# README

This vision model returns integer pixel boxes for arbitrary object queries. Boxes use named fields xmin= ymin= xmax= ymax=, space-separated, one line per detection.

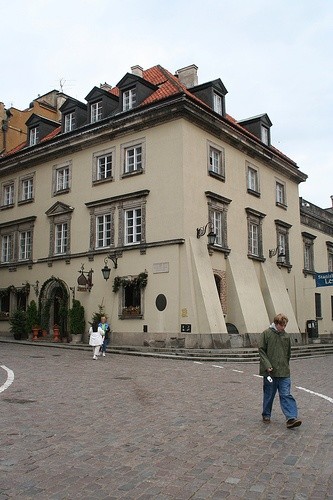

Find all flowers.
xmin=123 ymin=306 xmax=139 ymax=312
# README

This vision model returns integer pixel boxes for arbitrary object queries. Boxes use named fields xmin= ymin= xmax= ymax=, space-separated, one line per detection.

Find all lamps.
xmin=101 ymin=256 xmax=117 ymax=281
xmin=77 ymin=268 xmax=93 ymax=287
xmin=269 ymin=244 xmax=286 ymax=267
xmin=197 ymin=221 xmax=216 ymax=245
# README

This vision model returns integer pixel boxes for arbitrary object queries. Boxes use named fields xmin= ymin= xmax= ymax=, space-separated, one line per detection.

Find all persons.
xmin=98 ymin=316 xmax=108 ymax=356
xmin=89 ymin=321 xmax=105 ymax=360
xmin=257 ymin=314 xmax=302 ymax=428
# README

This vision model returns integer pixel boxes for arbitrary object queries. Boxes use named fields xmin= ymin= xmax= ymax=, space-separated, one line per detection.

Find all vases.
xmin=122 ymin=314 xmax=141 ymax=319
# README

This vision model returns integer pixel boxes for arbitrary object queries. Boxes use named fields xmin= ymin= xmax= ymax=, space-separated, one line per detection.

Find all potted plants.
xmin=68 ymin=299 xmax=86 ymax=342
xmin=26 ymin=299 xmax=37 ymax=340
xmin=9 ymin=309 xmax=27 ymax=341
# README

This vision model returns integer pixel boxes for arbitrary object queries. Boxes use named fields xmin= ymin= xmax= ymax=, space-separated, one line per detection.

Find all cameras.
xmin=267 ymin=376 xmax=273 ymax=382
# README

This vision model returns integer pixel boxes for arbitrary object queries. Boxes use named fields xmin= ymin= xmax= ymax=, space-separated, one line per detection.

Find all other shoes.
xmin=102 ymin=351 xmax=105 ymax=357
xmin=263 ymin=417 xmax=270 ymax=424
xmin=286 ymin=418 xmax=302 ymax=428
xmin=92 ymin=355 xmax=98 ymax=360
